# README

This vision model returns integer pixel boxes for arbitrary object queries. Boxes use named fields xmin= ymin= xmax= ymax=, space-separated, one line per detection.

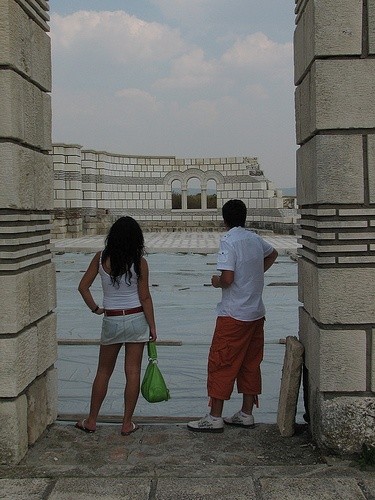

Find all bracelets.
xmin=91 ymin=306 xmax=99 ymax=313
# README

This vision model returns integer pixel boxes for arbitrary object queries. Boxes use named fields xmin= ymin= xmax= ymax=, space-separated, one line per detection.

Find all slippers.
xmin=121 ymin=422 xmax=139 ymax=435
xmin=75 ymin=419 xmax=95 ymax=433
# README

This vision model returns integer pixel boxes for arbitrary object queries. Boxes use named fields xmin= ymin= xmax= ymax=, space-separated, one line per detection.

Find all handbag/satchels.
xmin=141 ymin=341 xmax=170 ymax=403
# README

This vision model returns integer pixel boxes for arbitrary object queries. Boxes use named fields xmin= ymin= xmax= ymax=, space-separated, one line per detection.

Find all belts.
xmin=104 ymin=305 xmax=144 ymax=316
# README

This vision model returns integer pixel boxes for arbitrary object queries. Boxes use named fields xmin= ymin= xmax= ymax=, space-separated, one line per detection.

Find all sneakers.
xmin=187 ymin=416 xmax=224 ymax=432
xmin=223 ymin=412 xmax=255 ymax=429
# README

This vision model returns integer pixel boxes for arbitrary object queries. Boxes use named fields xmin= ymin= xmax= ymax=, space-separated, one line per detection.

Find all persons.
xmin=187 ymin=199 xmax=279 ymax=433
xmin=75 ymin=216 xmax=157 ymax=436
